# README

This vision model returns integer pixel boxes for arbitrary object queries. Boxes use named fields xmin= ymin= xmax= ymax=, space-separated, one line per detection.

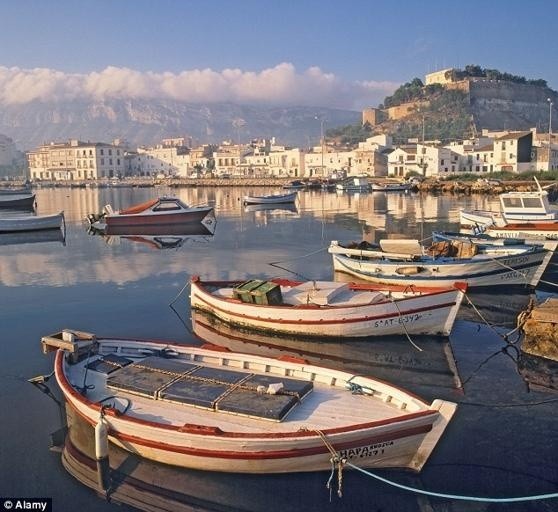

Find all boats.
xmin=242 ymin=189 xmax=297 ymax=206
xmin=86 ymin=197 xmax=221 ymax=237
xmin=0 ymin=186 xmax=63 ymax=233
xmin=2 ymin=229 xmax=67 ymax=249
xmin=108 ymin=233 xmax=210 ymax=252
xmin=328 ymin=229 xmax=555 ymax=291
xmin=38 ymin=329 xmax=457 ymax=479
xmin=280 ymin=174 xmax=413 ymax=191
xmin=187 ymin=310 xmax=489 ymax=391
xmin=179 ymin=276 xmax=467 ymax=337
xmin=34 ymin=379 xmax=305 ymax=506
xmin=245 ymin=204 xmax=300 ymax=215
xmin=455 ymin=285 xmax=540 ymax=328
xmin=457 ymin=190 xmax=557 ymax=235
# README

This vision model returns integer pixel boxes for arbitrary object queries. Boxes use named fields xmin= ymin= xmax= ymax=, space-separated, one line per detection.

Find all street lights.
xmin=546 ymin=96 xmax=554 ymax=169
xmin=414 ymin=106 xmax=426 ymax=178
xmin=313 ymin=114 xmax=324 ymax=180
